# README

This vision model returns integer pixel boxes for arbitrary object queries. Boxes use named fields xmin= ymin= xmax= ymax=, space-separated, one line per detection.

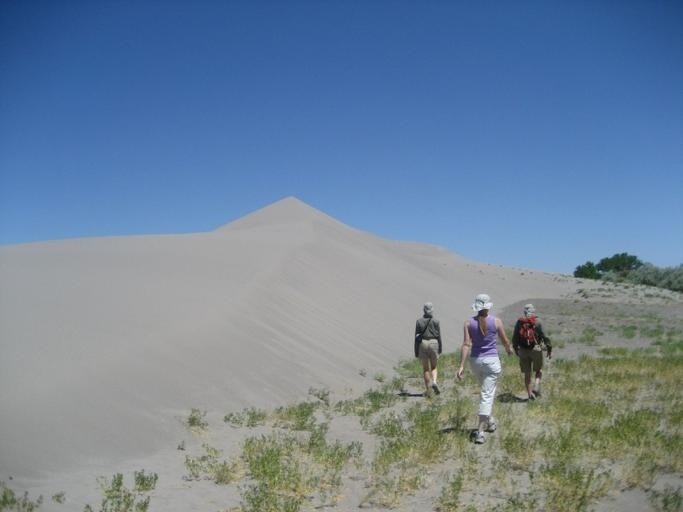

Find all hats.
xmin=470 ymin=293 xmax=495 ymax=312
xmin=422 ymin=302 xmax=436 ymax=313
xmin=522 ymin=302 xmax=537 ymax=316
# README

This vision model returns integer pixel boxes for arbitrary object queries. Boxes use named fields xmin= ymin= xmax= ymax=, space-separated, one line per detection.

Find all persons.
xmin=512 ymin=304 xmax=552 ymax=401
xmin=455 ymin=293 xmax=514 ymax=443
xmin=414 ymin=300 xmax=443 ymax=396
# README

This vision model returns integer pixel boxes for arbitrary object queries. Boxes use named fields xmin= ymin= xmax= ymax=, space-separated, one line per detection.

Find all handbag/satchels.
xmin=413 ymin=334 xmax=422 ymax=359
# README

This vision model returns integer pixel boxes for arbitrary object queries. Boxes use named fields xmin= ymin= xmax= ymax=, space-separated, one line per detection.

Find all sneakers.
xmin=474 ymin=431 xmax=487 ymax=444
xmin=423 ymin=382 xmax=440 ymax=400
xmin=487 ymin=420 xmax=498 ymax=433
xmin=528 ymin=386 xmax=542 ymax=400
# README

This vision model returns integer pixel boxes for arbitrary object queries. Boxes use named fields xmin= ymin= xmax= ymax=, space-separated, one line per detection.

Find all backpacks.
xmin=517 ymin=317 xmax=539 ymax=351
